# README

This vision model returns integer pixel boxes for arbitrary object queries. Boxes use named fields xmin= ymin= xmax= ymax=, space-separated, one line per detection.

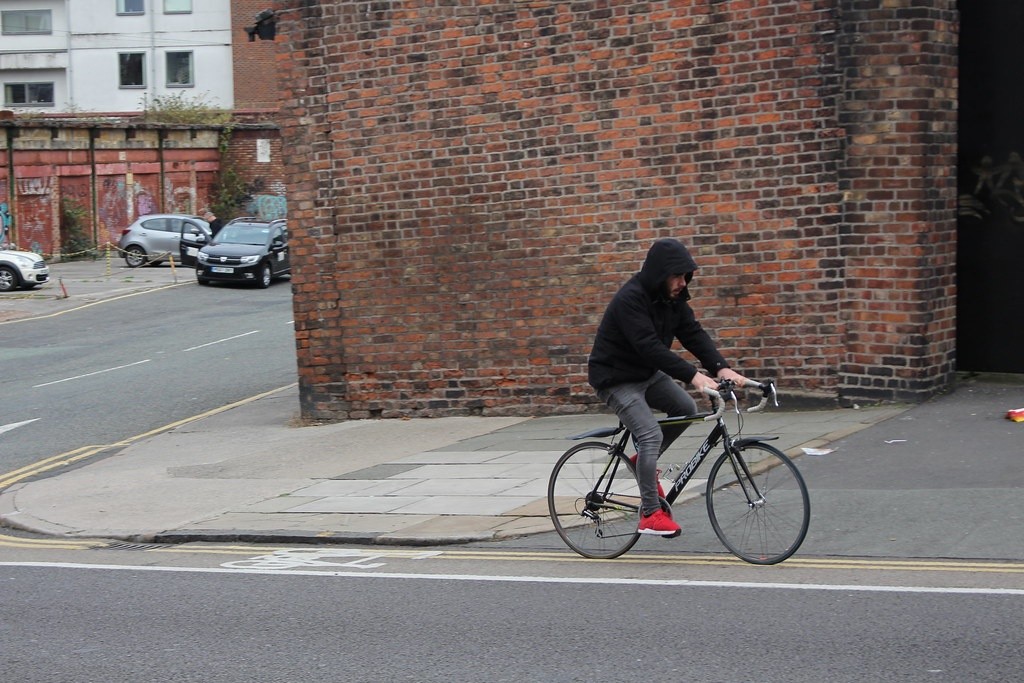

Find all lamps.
xmin=245 ymin=7 xmax=277 ymax=42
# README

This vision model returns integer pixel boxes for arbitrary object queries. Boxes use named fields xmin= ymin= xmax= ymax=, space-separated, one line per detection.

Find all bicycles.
xmin=547 ymin=374 xmax=811 ymax=565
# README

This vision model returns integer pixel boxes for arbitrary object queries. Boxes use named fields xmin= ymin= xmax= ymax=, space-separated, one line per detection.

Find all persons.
xmin=589 ymin=238 xmax=746 ymax=536
xmin=203 ymin=212 xmax=221 ymax=237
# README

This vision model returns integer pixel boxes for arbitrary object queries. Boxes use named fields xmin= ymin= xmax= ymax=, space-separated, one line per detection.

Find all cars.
xmin=0 ymin=248 xmax=51 ymax=293
xmin=117 ymin=212 xmax=214 ymax=269
xmin=179 ymin=216 xmax=291 ymax=289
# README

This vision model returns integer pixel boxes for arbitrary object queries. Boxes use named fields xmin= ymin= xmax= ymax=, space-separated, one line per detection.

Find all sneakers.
xmin=638 ymin=508 xmax=681 ymax=535
xmin=630 ymin=454 xmax=665 ymax=498
